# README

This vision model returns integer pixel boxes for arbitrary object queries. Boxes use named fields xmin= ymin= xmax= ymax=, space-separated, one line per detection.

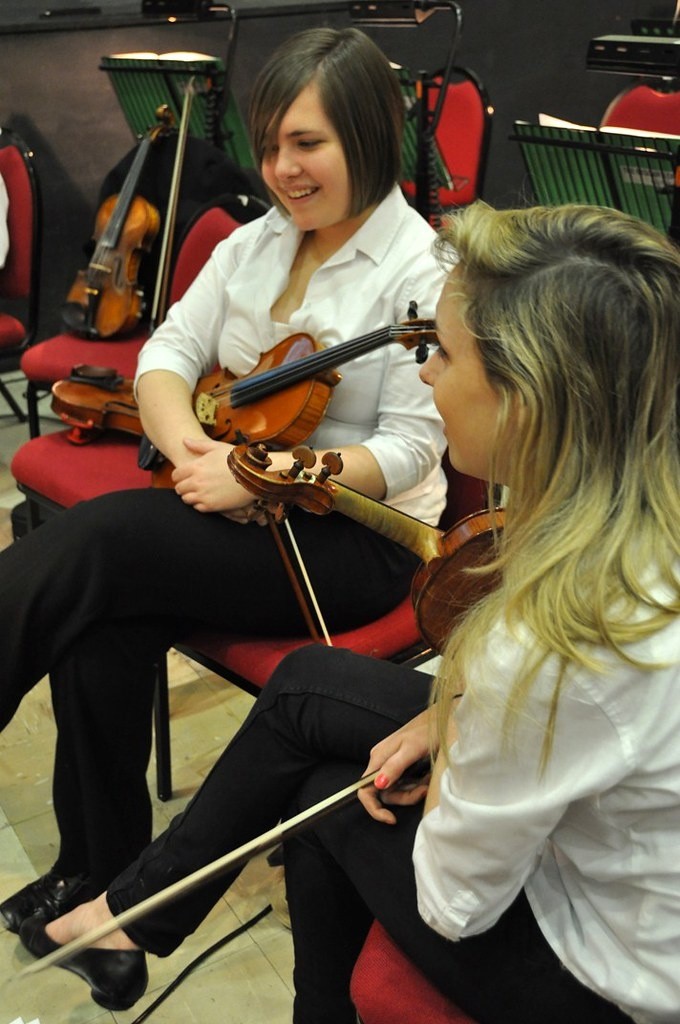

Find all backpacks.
xmin=100 ymin=134 xmax=261 ymax=333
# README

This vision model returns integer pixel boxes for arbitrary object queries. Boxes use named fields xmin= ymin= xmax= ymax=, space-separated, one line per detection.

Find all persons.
xmin=0 ymin=24 xmax=463 ymax=939
xmin=21 ymin=201 xmax=680 ymax=1024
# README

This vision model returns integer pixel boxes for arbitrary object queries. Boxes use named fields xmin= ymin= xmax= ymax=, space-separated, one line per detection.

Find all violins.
xmin=50 ymin=362 xmax=143 ymax=443
xmin=60 ymin=106 xmax=174 ymax=341
xmin=228 ymin=438 xmax=506 ymax=658
xmin=138 ymin=300 xmax=440 ymax=487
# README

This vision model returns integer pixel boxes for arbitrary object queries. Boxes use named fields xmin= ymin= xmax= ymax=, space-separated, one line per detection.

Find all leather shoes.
xmin=0 ymin=865 xmax=110 ymax=934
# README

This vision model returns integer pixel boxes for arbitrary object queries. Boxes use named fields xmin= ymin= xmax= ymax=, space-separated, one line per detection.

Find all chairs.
xmin=0 ymin=65 xmax=680 ymax=1024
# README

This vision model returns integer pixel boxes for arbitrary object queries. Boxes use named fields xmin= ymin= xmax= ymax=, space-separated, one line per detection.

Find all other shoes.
xmin=18 ymin=914 xmax=148 ymax=1011
